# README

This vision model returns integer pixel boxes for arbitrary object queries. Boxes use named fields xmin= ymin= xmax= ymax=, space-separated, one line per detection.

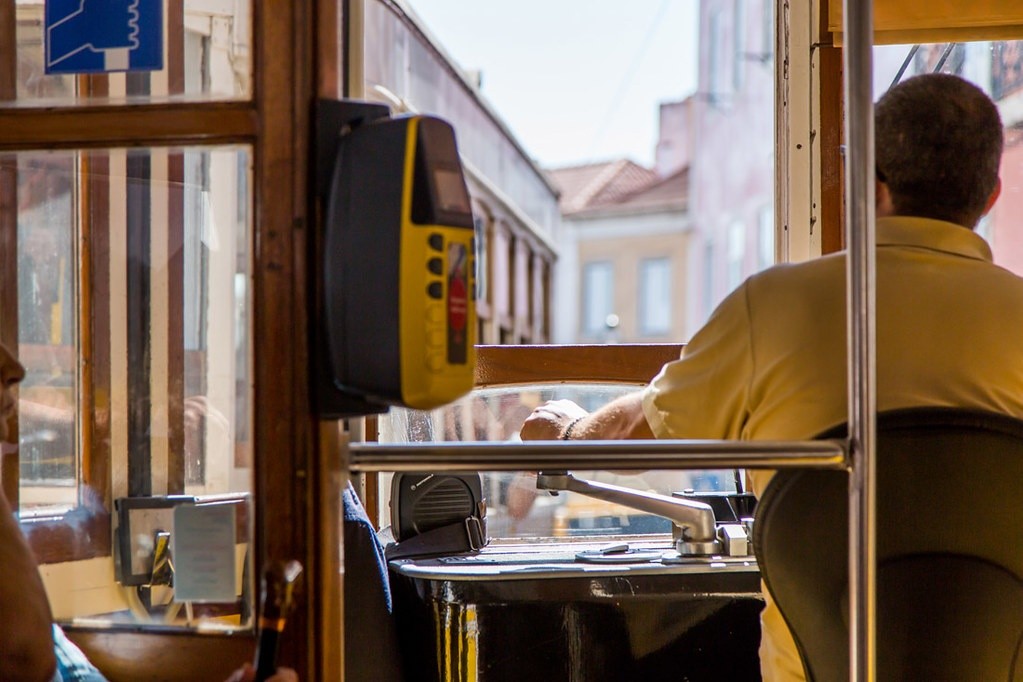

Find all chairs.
xmin=751 ymin=407 xmax=1023 ymax=682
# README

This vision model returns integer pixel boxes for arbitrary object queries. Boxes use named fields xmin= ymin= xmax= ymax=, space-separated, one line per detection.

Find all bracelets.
xmin=563 ymin=417 xmax=582 ymax=439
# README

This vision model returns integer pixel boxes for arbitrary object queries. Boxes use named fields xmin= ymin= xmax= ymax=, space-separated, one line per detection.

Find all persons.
xmin=507 ymin=410 xmax=692 ymax=535
xmin=0 ymin=342 xmax=107 ymax=682
xmin=520 ymin=73 xmax=1023 ymax=682
